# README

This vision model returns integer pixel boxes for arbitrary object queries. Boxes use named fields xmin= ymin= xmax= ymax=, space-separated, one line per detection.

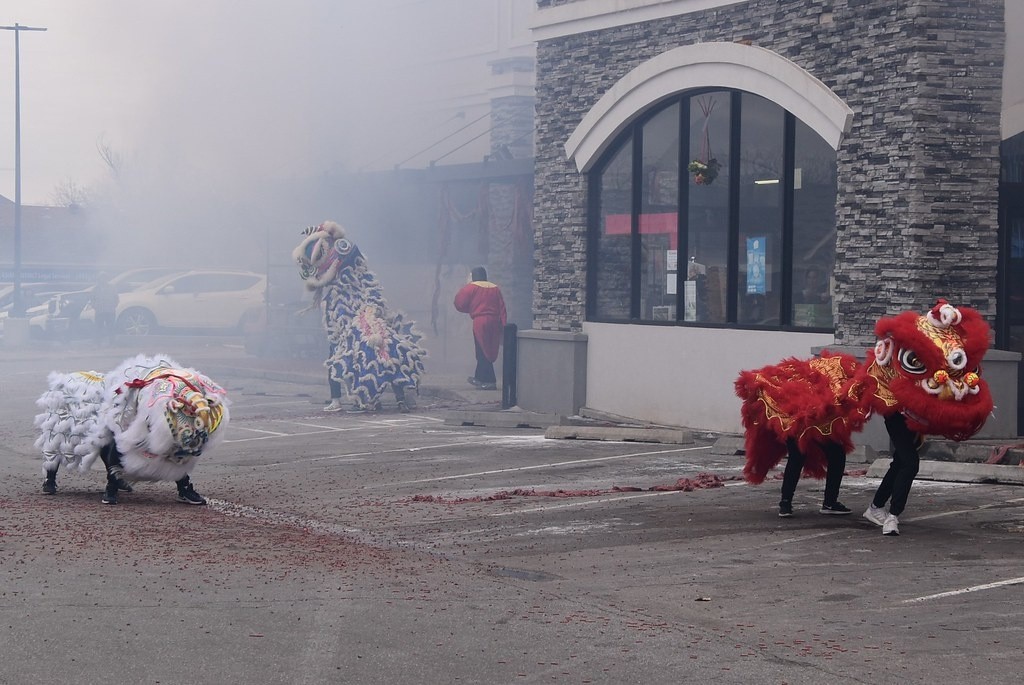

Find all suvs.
xmin=80 ymin=268 xmax=270 ymax=335
xmin=50 ymin=267 xmax=161 ymax=317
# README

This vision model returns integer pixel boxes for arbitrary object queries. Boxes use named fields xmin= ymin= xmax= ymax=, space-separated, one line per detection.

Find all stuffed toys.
xmin=734 ymin=298 xmax=994 ymax=487
xmin=34 ymin=353 xmax=231 ymax=486
xmin=292 ymin=220 xmax=429 ymax=410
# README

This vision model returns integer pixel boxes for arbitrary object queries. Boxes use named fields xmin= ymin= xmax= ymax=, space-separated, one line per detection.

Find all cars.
xmin=35 ymin=291 xmax=108 ymax=339
xmin=0 ymin=281 xmax=86 ymax=341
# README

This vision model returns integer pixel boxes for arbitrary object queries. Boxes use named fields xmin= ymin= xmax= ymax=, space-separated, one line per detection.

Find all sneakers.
xmin=41 ymin=478 xmax=56 ymax=496
xmin=324 ymin=401 xmax=342 ymax=413
xmin=348 ymin=399 xmax=385 ymax=412
xmin=862 ymin=504 xmax=900 ymax=536
xmin=102 ymin=477 xmax=133 ymax=505
xmin=176 ymin=482 xmax=205 ymax=505
xmin=819 ymin=501 xmax=854 ymax=514
xmin=778 ymin=500 xmax=792 ymax=518
xmin=395 ymin=400 xmax=409 ymax=413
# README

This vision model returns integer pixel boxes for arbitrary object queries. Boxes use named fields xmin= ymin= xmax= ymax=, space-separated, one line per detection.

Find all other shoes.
xmin=467 ymin=376 xmax=498 ymax=390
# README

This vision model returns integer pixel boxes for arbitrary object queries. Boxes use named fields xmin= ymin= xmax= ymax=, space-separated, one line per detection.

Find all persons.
xmin=21 ymin=289 xmax=41 ymax=311
xmin=797 ymin=265 xmax=830 ymax=304
xmin=453 ymin=267 xmax=509 ymax=389
xmin=101 ymin=438 xmax=207 ymax=504
xmin=88 ymin=271 xmax=119 ymax=349
xmin=347 ymin=385 xmax=411 ymax=412
xmin=862 ymin=411 xmax=924 ymax=535
xmin=324 ymin=343 xmax=382 ymax=411
xmin=778 ymin=434 xmax=852 ymax=516
xmin=42 ymin=442 xmax=132 ymax=494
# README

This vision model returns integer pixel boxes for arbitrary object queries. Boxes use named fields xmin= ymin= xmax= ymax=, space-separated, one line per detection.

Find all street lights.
xmin=0 ymin=23 xmax=54 ymax=321
